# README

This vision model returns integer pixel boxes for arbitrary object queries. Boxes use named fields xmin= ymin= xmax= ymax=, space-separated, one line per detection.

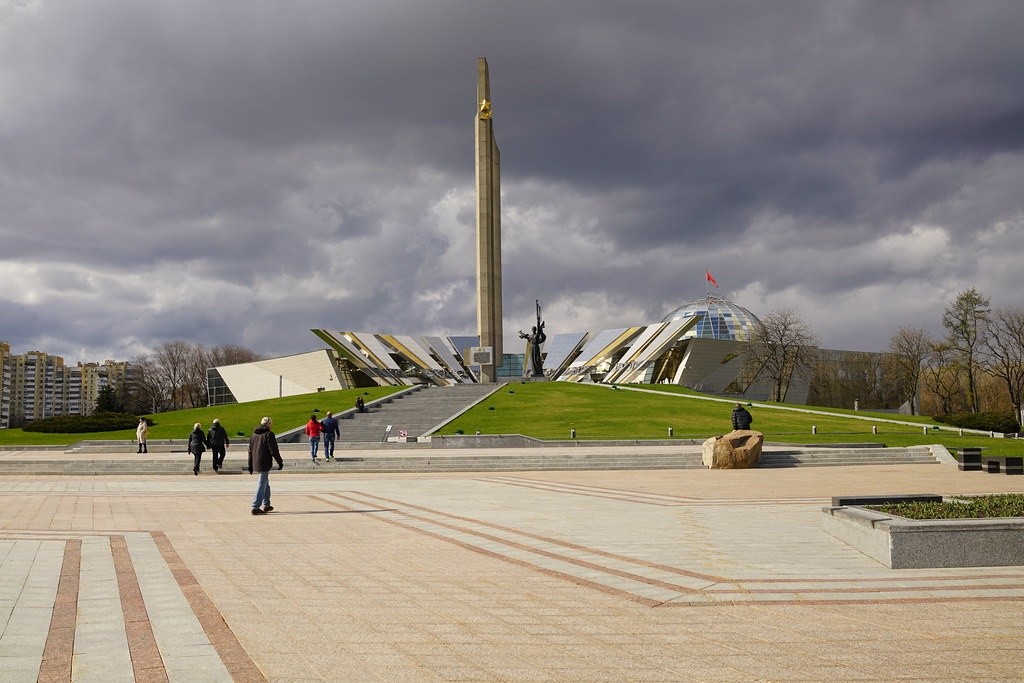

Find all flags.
xmin=705 ymin=271 xmax=719 ymax=288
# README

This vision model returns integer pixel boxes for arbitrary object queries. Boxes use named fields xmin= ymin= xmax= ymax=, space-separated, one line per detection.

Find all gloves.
xmin=277 ymin=463 xmax=283 ymax=470
xmin=247 ymin=467 xmax=254 ymax=475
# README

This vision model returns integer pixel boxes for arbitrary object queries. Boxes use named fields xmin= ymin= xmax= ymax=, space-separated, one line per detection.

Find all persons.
xmin=320 ymin=411 xmax=340 ymax=462
xmin=305 ymin=414 xmax=324 ymax=461
xmin=355 ymin=396 xmax=364 ymax=412
xmin=523 ymin=326 xmax=543 ymax=374
xmin=247 ymin=416 xmax=284 ymax=515
xmin=731 ymin=402 xmax=753 ymax=430
xmin=207 ymin=418 xmax=229 ymax=470
xmin=187 ymin=423 xmax=207 ymax=476
xmin=136 ymin=417 xmax=148 ymax=453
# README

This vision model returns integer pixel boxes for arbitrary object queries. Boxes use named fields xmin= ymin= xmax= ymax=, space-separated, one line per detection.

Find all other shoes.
xmin=312 ymin=458 xmax=315 ymax=461
xmin=326 ymin=458 xmax=329 ymax=462
xmin=137 ymin=450 xmax=142 ymax=453
xmin=143 ymin=450 xmax=147 ymax=453
xmin=214 ymin=470 xmax=219 ymax=474
xmin=193 ymin=468 xmax=202 ymax=476
xmin=330 ymin=456 xmax=334 ymax=459
xmin=314 ymin=454 xmax=317 ymax=457
xmin=217 ymin=463 xmax=223 ymax=469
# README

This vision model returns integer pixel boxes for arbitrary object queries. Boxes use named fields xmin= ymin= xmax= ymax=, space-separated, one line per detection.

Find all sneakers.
xmin=264 ymin=506 xmax=274 ymax=511
xmin=251 ymin=508 xmax=267 ymax=515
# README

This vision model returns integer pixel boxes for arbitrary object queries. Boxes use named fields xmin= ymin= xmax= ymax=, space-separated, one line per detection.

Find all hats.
xmin=194 ymin=423 xmax=201 ymax=428
xmin=213 ymin=418 xmax=219 ymax=424
xmin=260 ymin=417 xmax=271 ymax=426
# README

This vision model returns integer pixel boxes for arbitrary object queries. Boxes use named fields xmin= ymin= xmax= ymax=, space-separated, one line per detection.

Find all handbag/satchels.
xmin=201 ymin=443 xmax=206 ymax=452
